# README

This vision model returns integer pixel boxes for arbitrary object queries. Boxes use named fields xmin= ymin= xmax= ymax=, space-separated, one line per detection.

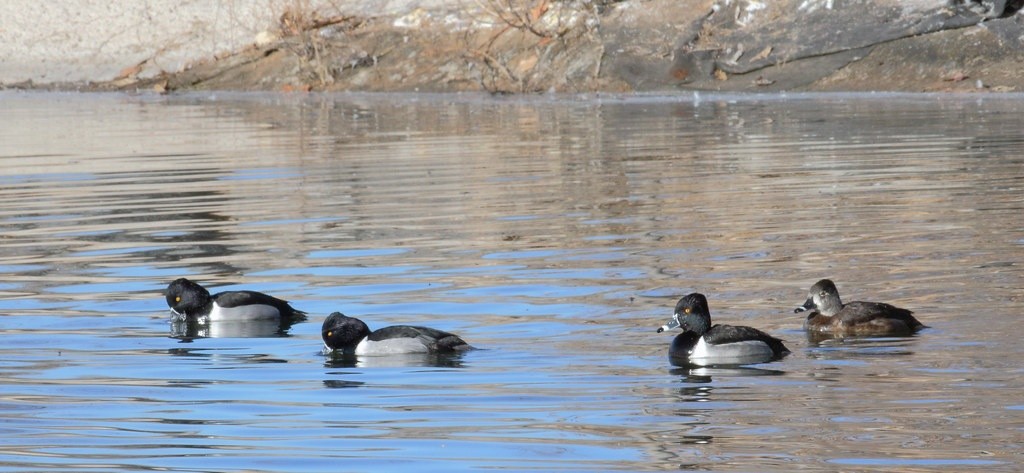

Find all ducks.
xmin=795 ymin=279 xmax=936 ymax=334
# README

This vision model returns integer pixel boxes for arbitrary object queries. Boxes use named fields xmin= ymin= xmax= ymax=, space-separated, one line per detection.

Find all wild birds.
xmin=166 ymin=278 xmax=308 ymax=322
xmin=657 ymin=293 xmax=791 ymax=359
xmin=321 ymin=312 xmax=477 ymax=355
